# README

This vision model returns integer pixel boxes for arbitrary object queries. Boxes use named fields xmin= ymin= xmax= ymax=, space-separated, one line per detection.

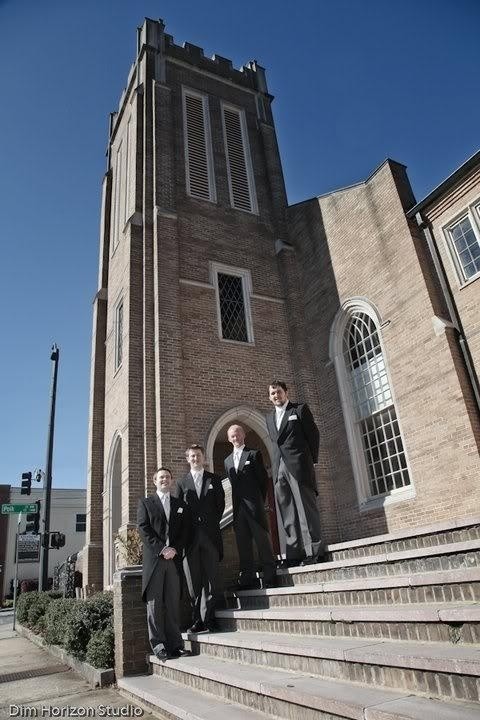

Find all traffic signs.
xmin=1 ymin=503 xmax=38 ymax=514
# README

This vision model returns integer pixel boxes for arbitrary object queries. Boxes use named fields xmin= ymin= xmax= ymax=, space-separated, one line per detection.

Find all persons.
xmin=137 ymin=467 xmax=191 ymax=662
xmin=263 ymin=377 xmax=326 ymax=570
xmin=223 ymin=421 xmax=281 ymax=592
xmin=175 ymin=443 xmax=226 ymax=635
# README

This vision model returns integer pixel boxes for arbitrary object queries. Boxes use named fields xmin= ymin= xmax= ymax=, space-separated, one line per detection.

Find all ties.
xmin=276 ymin=410 xmax=284 ymax=431
xmin=194 ymin=472 xmax=203 ymax=499
xmin=162 ymin=495 xmax=170 ymax=522
xmin=234 ymin=451 xmax=240 ymax=475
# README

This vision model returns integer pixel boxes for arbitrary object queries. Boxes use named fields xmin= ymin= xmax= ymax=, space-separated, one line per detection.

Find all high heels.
xmin=156 ymin=646 xmax=194 ymax=662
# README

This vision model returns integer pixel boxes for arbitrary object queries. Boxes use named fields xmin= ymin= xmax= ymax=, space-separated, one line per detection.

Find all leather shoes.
xmin=282 ymin=551 xmax=329 ymax=568
xmin=187 ymin=619 xmax=209 ymax=634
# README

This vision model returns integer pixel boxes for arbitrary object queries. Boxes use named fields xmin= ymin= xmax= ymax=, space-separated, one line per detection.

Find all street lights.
xmin=38 ymin=342 xmax=59 ymax=592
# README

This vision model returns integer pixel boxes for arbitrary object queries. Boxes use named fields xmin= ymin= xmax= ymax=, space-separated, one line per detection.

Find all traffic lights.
xmin=25 ymin=500 xmax=40 ymax=531
xmin=20 ymin=471 xmax=31 ymax=495
